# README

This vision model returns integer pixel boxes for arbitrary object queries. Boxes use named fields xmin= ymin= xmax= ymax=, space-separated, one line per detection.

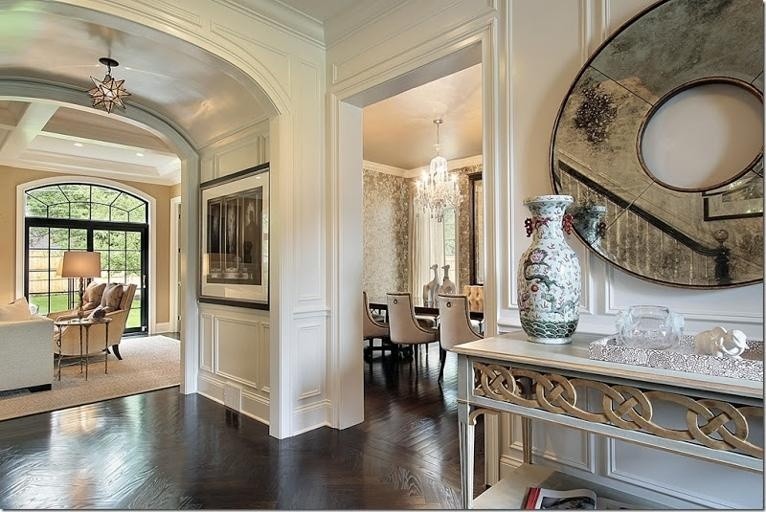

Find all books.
xmin=524 ymin=486 xmax=632 ymax=510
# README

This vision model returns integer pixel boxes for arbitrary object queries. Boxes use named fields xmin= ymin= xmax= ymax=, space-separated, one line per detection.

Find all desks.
xmin=54 ymin=318 xmax=113 ymax=380
xmin=447 ymin=330 xmax=765 ymax=511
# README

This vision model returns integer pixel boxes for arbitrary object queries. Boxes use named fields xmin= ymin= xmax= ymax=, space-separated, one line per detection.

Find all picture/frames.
xmin=197 ymin=161 xmax=271 ymax=310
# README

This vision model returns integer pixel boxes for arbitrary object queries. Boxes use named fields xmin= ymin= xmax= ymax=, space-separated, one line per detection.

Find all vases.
xmin=517 ymin=195 xmax=581 ymax=344
xmin=574 ymin=205 xmax=608 ymax=258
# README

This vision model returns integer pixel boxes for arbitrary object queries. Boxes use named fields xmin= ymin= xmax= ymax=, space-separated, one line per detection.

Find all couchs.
xmin=53 ymin=281 xmax=137 ymax=360
xmin=0 ymin=318 xmax=55 ymax=393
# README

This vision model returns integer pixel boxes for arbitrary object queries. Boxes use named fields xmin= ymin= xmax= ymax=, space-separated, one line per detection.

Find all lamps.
xmin=61 ymin=252 xmax=101 ymax=318
xmin=413 ymin=119 xmax=463 ymax=222
xmin=87 ymin=57 xmax=131 ymax=113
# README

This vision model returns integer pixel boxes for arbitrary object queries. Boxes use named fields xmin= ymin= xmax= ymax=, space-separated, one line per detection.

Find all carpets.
xmin=0 ymin=334 xmax=181 ymax=422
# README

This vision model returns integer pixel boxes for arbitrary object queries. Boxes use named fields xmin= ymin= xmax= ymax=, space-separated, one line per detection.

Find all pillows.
xmin=0 ymin=298 xmax=31 ymax=321
xmin=77 ymin=282 xmax=124 ymax=316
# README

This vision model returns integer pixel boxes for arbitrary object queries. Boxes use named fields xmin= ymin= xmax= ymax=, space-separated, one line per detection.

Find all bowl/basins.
xmin=620 ymin=305 xmax=677 ymax=349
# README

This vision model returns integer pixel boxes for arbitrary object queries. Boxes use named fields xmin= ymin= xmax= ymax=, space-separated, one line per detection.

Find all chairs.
xmin=363 ymin=284 xmax=484 ymax=382
